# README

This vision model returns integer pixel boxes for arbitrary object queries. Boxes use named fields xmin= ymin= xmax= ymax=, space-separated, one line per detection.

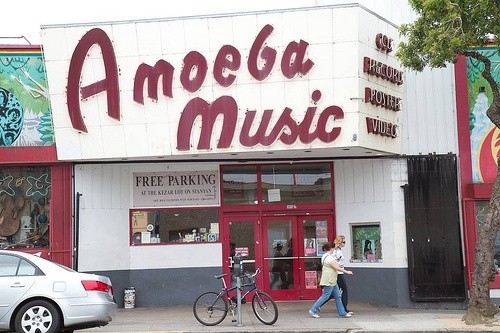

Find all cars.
xmin=0 ymin=247 xmax=119 ymax=333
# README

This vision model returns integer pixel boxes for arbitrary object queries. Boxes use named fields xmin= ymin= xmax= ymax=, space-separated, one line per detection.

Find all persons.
xmin=268 ymin=241 xmax=288 ymax=289
xmin=307 ymin=241 xmax=354 ymax=319
xmin=277 ymin=237 xmax=295 ymax=289
xmin=314 ymin=235 xmax=353 ymax=313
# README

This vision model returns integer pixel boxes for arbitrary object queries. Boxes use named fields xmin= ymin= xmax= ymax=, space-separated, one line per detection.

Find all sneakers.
xmin=309 ymin=304 xmax=354 ymax=318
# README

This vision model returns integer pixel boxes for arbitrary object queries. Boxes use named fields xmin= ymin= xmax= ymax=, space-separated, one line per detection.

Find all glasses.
xmin=331 ymin=243 xmax=334 ymax=247
xmin=341 ymin=241 xmax=346 ymax=244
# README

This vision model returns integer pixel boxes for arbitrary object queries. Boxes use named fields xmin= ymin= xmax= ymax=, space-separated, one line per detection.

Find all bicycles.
xmin=193 ymin=264 xmax=279 ymax=327
